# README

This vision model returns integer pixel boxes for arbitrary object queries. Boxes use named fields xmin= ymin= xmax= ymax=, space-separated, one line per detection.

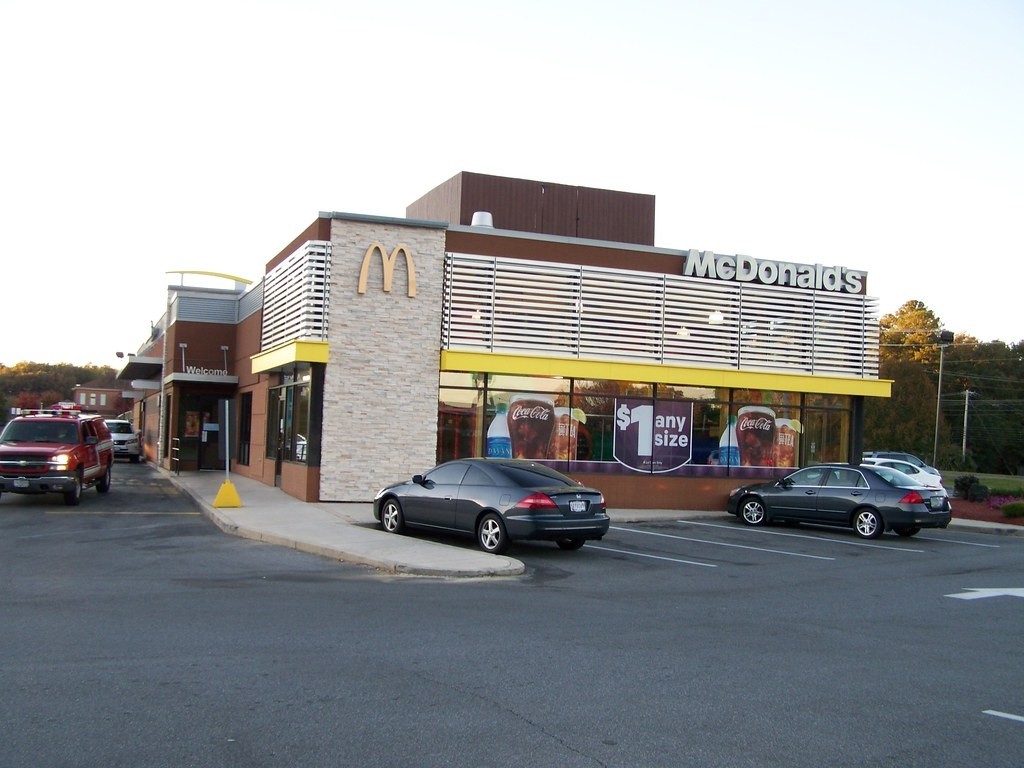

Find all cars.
xmin=372 ymin=456 xmax=610 ymax=556
xmin=727 ymin=465 xmax=953 ymax=539
xmin=864 ymin=452 xmax=941 ymax=477
xmin=862 ymin=458 xmax=950 ymax=499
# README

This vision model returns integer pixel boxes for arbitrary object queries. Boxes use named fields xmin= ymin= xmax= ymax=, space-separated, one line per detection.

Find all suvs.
xmin=0 ymin=405 xmax=115 ymax=506
xmin=104 ymin=419 xmax=142 ymax=463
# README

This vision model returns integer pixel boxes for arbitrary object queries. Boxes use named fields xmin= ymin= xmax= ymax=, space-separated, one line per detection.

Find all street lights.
xmin=932 ymin=331 xmax=956 ymax=464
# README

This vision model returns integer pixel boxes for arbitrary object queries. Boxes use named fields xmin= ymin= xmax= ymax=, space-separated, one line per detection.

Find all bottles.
xmin=719 ymin=415 xmax=741 ymax=467
xmin=486 ymin=403 xmax=512 ymax=459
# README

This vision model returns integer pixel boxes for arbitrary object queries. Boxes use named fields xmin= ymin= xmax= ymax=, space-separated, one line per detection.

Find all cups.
xmin=735 ymin=406 xmax=775 ymax=467
xmin=548 ymin=407 xmax=580 ymax=461
xmin=775 ymin=418 xmax=801 ymax=468
xmin=506 ymin=393 xmax=554 ymax=459
